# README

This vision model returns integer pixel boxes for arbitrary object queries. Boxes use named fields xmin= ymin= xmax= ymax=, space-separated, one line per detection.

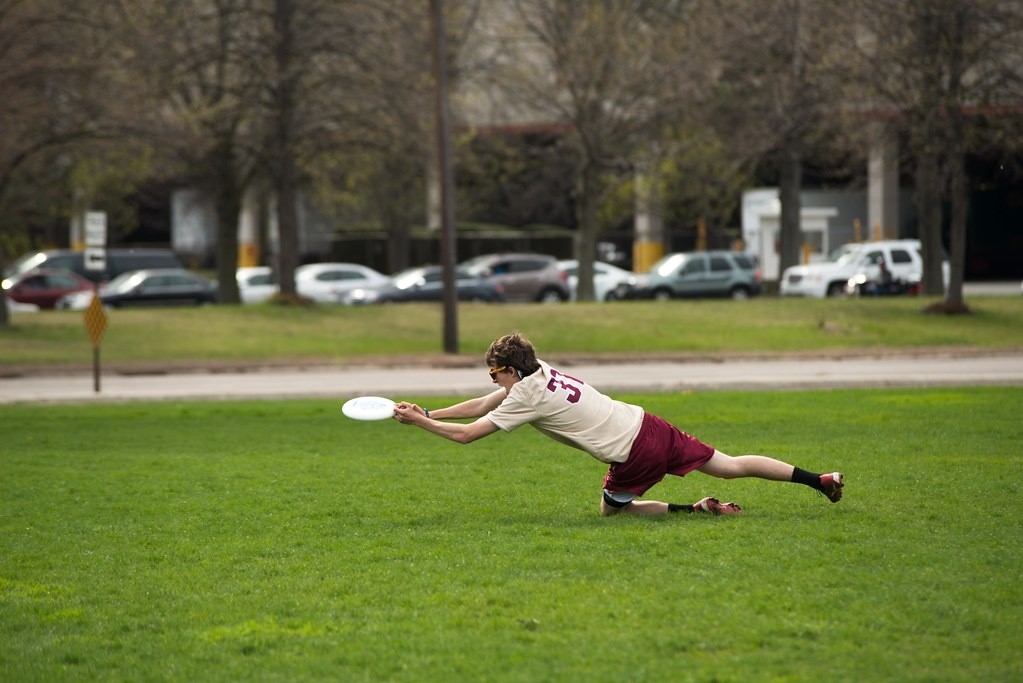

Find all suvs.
xmin=615 ymin=251 xmax=760 ymax=301
xmin=780 ymin=237 xmax=952 ymax=298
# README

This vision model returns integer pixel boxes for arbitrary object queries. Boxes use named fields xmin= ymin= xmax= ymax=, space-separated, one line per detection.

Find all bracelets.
xmin=424 ymin=409 xmax=430 ymax=417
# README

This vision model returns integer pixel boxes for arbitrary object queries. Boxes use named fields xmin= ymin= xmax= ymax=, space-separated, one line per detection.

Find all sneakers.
xmin=816 ymin=472 xmax=845 ymax=503
xmin=693 ymin=497 xmax=741 ymax=515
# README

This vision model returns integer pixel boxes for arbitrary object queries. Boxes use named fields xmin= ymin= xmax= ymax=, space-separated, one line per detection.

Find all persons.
xmin=392 ymin=333 xmax=843 ymax=515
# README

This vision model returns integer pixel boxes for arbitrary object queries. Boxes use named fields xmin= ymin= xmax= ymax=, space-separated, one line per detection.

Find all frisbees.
xmin=341 ymin=395 xmax=398 ymax=422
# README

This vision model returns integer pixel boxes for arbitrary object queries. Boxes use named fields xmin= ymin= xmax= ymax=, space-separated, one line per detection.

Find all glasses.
xmin=489 ymin=366 xmax=506 ymax=380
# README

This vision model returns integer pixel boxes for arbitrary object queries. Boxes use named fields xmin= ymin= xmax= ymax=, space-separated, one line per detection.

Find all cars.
xmin=0 ymin=245 xmax=637 ymax=312
